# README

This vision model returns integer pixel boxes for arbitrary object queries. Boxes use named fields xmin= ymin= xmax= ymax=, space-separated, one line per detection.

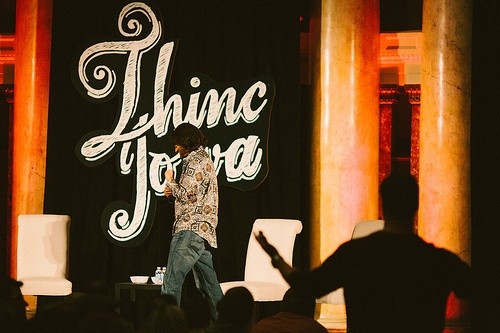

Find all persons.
xmin=0 ymin=268 xmax=333 ymax=333
xmin=159 ymin=122 xmax=226 ymax=333
xmin=256 ymin=173 xmax=499 ymax=333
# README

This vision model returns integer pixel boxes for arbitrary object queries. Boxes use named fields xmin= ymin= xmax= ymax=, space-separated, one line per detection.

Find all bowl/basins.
xmin=151 ymin=277 xmax=159 ymax=284
xmin=130 ymin=276 xmax=149 ymax=284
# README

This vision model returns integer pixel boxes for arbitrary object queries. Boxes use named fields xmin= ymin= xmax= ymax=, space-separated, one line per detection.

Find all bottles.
xmin=155 ymin=267 xmax=161 ymax=279
xmin=161 ymin=267 xmax=166 ymax=284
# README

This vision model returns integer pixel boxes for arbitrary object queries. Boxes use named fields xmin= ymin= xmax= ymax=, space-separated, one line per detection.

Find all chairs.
xmin=322 ymin=221 xmax=389 ymax=304
xmin=17 ymin=215 xmax=72 ymax=295
xmin=218 ymin=218 xmax=302 ymax=302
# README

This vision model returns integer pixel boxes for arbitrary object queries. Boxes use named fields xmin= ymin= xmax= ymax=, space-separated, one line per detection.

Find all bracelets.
xmin=271 ymin=255 xmax=282 ymax=268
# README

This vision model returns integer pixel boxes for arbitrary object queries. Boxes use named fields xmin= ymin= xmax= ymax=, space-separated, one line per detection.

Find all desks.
xmin=115 ymin=284 xmax=170 ymax=312
